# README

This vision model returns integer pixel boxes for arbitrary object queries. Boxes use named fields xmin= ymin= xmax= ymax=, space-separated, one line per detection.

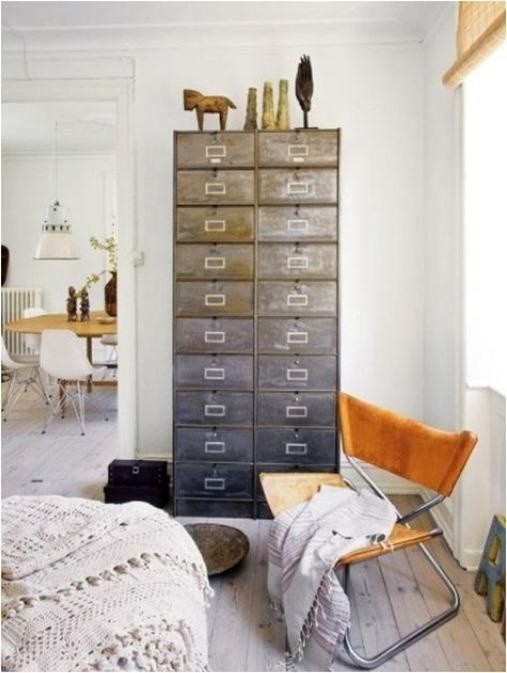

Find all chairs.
xmin=258 ymin=389 xmax=478 ymax=668
xmin=2 ymin=307 xmax=117 ymax=435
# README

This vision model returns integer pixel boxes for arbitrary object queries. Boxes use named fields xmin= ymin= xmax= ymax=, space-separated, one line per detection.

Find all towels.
xmin=267 ymin=486 xmax=395 ymax=669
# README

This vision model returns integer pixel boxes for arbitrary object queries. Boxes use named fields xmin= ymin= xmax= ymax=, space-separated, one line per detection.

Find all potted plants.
xmin=74 ymin=215 xmax=117 ymax=316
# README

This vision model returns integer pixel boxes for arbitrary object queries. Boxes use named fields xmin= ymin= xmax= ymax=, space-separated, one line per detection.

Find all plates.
xmin=95 ymin=316 xmax=114 ymax=324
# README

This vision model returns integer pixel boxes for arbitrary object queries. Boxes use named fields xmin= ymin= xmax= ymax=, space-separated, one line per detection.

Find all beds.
xmin=1 ymin=492 xmax=212 ymax=671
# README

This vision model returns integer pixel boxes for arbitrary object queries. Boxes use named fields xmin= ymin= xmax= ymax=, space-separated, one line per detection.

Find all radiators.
xmin=2 ymin=287 xmax=41 ymax=356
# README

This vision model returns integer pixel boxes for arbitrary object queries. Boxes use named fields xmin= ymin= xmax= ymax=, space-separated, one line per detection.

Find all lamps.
xmin=34 ymin=120 xmax=80 ymax=260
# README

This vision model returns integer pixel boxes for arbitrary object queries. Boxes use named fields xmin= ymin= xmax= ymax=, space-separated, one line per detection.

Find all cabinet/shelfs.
xmin=173 ymin=129 xmax=339 ymax=517
